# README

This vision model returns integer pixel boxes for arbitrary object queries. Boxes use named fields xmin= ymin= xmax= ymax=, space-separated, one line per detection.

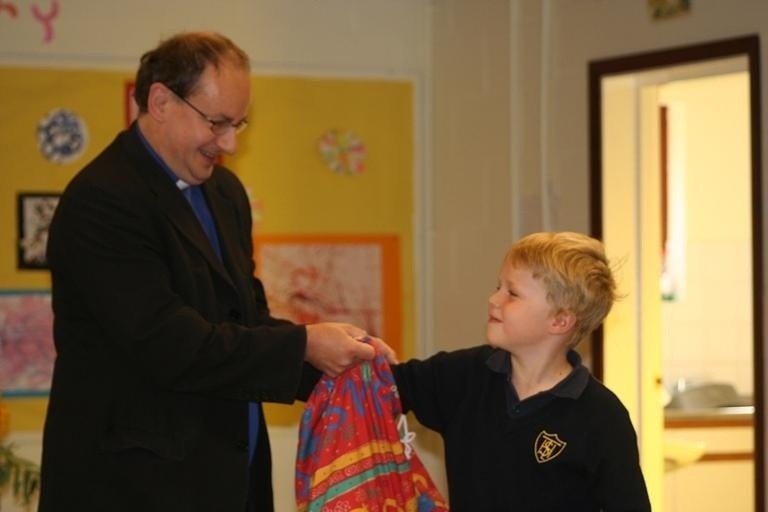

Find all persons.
xmin=378 ymin=227 xmax=653 ymax=510
xmin=37 ymin=30 xmax=404 ymax=512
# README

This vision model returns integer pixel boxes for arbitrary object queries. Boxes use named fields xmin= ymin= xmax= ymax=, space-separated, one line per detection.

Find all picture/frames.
xmin=13 ymin=190 xmax=61 ymax=272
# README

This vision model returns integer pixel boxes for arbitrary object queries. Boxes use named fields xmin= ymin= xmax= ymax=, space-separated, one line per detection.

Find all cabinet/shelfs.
xmin=666 ymin=410 xmax=756 ymax=512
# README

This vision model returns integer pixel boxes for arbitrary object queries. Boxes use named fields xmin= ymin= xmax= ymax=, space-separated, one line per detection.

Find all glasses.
xmin=162 ymin=83 xmax=249 ymax=136
